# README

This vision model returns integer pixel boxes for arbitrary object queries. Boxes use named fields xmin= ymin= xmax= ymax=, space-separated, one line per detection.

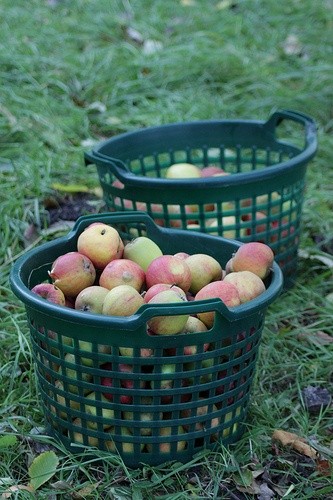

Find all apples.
xmin=110 ymin=163 xmax=300 ymax=272
xmin=26 ymin=222 xmax=273 ymax=455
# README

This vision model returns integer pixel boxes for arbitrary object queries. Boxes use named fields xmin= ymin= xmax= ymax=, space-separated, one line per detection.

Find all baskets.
xmin=10 ymin=211 xmax=283 ymax=471
xmin=82 ymin=108 xmax=318 ymax=301
